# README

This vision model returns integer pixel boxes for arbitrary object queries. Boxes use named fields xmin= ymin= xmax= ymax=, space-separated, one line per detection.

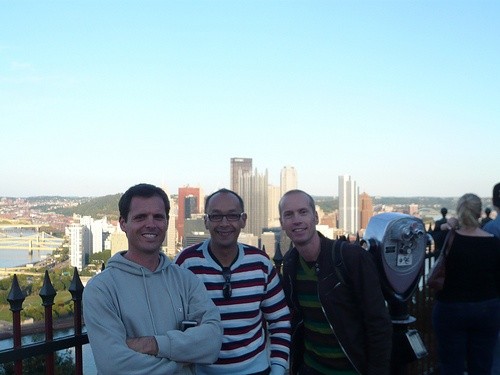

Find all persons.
xmin=172 ymin=189 xmax=292 ymax=375
xmin=425 ymin=183 xmax=500 ymax=375
xmin=272 ymin=190 xmax=392 ymax=374
xmin=82 ymin=183 xmax=224 ymax=374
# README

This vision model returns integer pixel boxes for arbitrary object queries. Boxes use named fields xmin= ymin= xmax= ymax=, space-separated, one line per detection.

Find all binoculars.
xmin=366 ymin=213 xmax=429 ymax=296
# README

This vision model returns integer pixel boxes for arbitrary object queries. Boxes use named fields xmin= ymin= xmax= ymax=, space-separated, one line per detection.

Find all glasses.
xmin=207 ymin=213 xmax=243 ymax=222
xmin=222 ymin=267 xmax=232 ymax=299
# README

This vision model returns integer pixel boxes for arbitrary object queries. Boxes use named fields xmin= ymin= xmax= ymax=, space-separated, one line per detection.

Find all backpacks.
xmin=284 ymin=239 xmax=392 ymax=300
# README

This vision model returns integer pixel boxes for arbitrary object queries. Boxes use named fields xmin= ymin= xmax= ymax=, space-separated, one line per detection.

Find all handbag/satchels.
xmin=428 ymin=229 xmax=458 ymax=296
